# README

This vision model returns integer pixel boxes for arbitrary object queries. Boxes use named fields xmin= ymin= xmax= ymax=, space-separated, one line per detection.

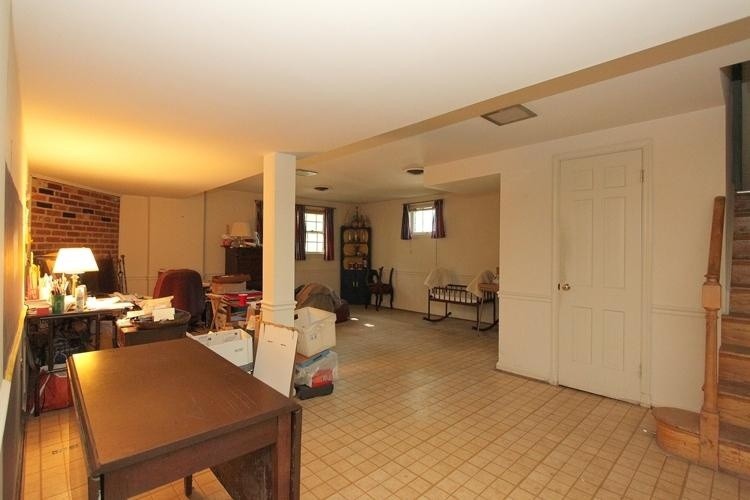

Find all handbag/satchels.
xmin=27 ymin=353 xmax=75 ymax=413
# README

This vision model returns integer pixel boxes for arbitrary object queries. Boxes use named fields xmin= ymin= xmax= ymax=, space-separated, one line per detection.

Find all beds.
xmin=422 ymin=266 xmax=499 ymax=331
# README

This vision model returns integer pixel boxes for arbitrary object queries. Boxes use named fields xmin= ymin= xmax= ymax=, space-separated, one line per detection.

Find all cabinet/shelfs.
xmin=340 ymin=225 xmax=372 ymax=305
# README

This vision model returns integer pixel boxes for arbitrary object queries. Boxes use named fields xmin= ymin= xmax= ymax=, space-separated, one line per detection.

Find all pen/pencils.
xmin=51 ymin=273 xmax=69 ymax=295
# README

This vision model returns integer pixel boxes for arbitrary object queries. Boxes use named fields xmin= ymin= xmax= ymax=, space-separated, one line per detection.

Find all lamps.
xmin=229 ymin=221 xmax=254 ymax=248
xmin=51 ymin=247 xmax=99 ymax=299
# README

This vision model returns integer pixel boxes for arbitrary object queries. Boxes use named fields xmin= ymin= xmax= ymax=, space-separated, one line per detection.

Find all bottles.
xmin=74 ymin=286 xmax=85 ymax=312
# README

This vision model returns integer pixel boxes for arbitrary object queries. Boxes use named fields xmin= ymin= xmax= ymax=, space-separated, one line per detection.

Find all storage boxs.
xmin=186 ymin=328 xmax=254 ymax=375
xmin=293 ymin=307 xmax=338 ymax=357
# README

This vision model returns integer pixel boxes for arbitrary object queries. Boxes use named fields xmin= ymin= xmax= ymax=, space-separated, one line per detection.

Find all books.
xmin=219 ymin=288 xmax=262 ymax=320
xmin=26 ymin=292 xmax=176 ymax=328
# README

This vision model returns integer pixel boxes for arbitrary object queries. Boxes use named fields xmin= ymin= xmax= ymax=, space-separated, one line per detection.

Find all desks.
xmin=64 ymin=338 xmax=302 ymax=500
xmin=26 ymin=298 xmax=127 ymax=418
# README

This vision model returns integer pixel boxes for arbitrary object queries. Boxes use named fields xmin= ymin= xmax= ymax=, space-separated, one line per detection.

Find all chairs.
xmin=152 ymin=269 xmax=205 ymax=327
xmin=364 ymin=269 xmax=394 ymax=312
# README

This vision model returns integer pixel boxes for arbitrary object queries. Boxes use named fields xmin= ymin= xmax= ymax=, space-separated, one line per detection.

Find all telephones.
xmin=108 ymin=292 xmax=139 ymax=301
